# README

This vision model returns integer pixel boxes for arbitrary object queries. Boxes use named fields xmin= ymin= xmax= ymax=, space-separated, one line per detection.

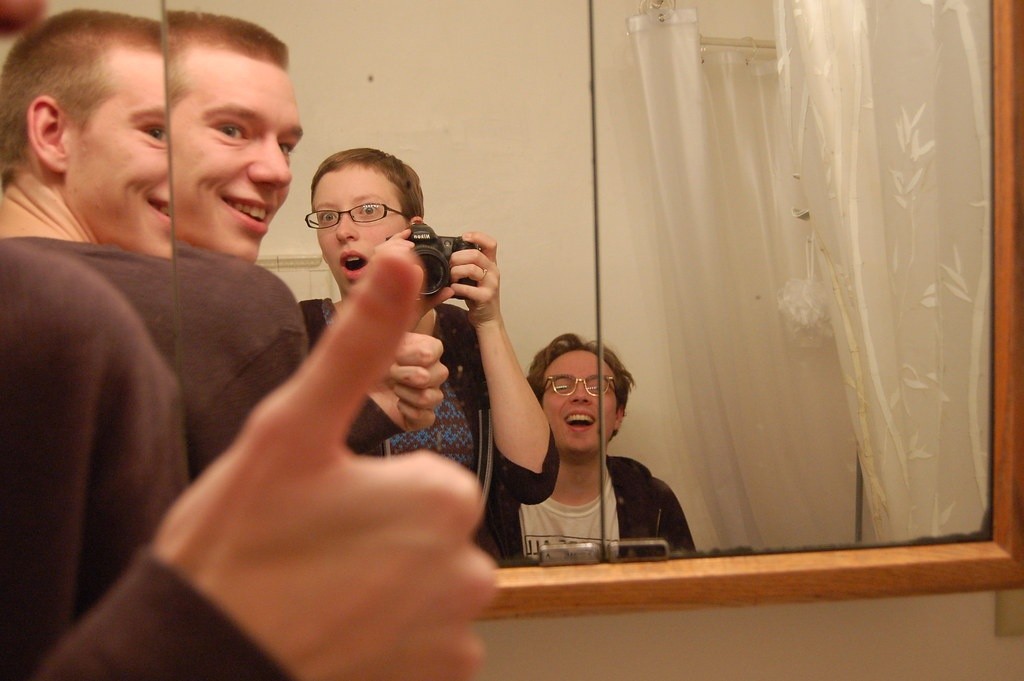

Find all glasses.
xmin=304 ymin=203 xmax=405 ymax=229
xmin=542 ymin=374 xmax=616 ymax=396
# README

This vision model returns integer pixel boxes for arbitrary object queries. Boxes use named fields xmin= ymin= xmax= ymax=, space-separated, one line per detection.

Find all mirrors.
xmin=161 ymin=0 xmax=995 ymax=567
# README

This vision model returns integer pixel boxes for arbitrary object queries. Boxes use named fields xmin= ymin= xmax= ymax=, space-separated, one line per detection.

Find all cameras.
xmin=386 ymin=223 xmax=480 ymax=300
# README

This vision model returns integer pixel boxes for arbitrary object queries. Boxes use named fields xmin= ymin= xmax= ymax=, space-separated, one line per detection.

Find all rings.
xmin=479 ymin=268 xmax=488 ymax=281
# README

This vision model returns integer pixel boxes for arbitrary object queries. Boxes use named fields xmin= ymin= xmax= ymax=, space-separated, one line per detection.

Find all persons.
xmin=1 ymin=7 xmax=178 ymax=366
xmin=168 ymin=8 xmax=311 ymax=480
xmin=0 ymin=238 xmax=498 ymax=681
xmin=505 ymin=333 xmax=695 ymax=565
xmin=296 ymin=148 xmax=561 ymax=568
